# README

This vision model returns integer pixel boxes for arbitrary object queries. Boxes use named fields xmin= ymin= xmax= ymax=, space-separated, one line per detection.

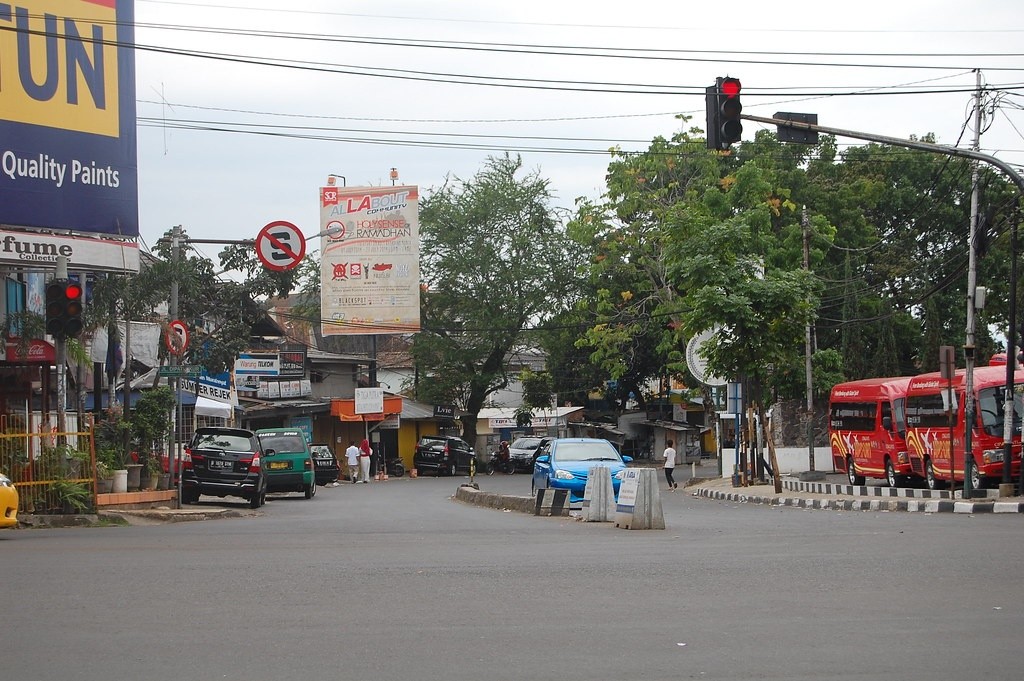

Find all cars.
xmin=308 ymin=441 xmax=341 ymax=486
xmin=530 ymin=438 xmax=635 ymax=504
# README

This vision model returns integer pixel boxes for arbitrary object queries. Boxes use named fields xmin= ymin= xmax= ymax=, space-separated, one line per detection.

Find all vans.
xmin=828 ymin=375 xmax=915 ymax=486
xmin=252 ymin=427 xmax=318 ymax=498
xmin=508 ymin=435 xmax=557 ymax=472
xmin=903 ymin=358 xmax=1023 ymax=489
xmin=413 ymin=435 xmax=478 ymax=478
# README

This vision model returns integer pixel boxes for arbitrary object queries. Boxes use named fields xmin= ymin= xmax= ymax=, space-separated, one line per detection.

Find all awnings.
xmin=329 ymin=396 xmax=403 ymax=415
xmin=0 ymin=335 xmax=57 ymax=453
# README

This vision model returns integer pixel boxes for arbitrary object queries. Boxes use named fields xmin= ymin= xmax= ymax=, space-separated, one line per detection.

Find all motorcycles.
xmin=378 ymin=456 xmax=405 ymax=477
xmin=485 ymin=449 xmax=516 ymax=476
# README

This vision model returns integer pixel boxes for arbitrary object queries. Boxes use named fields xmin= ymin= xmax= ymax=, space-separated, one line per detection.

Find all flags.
xmin=103 ymin=319 xmax=125 ymax=382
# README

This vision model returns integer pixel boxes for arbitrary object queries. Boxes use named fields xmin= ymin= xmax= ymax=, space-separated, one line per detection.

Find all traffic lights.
xmin=61 ymin=279 xmax=83 ymax=338
xmin=44 ymin=281 xmax=64 ymax=337
xmin=714 ymin=76 xmax=745 ymax=143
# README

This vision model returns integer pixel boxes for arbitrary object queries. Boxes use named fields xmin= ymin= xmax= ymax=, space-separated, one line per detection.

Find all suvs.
xmin=180 ymin=426 xmax=276 ymax=509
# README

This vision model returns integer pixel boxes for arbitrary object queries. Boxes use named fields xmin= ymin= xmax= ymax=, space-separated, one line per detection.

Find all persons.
xmin=498 ymin=440 xmax=510 ymax=463
xmin=345 ymin=439 xmax=361 ymax=483
xmin=662 ymin=438 xmax=678 ymax=490
xmin=359 ymin=439 xmax=374 ymax=482
